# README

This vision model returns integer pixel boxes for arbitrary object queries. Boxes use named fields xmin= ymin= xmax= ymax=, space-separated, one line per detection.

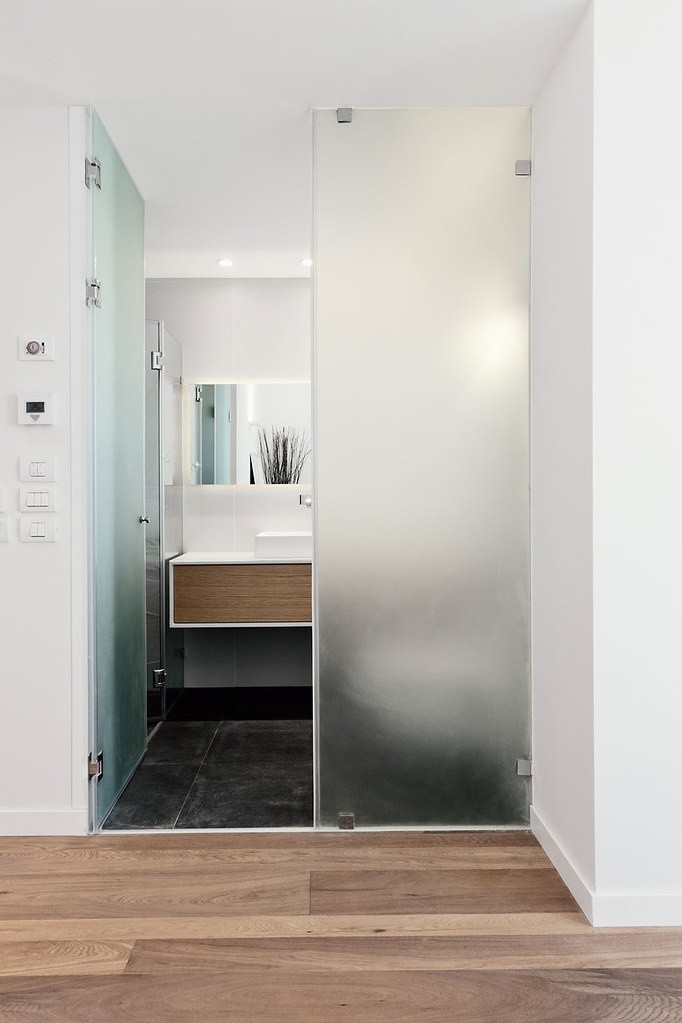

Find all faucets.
xmin=300 ymin=494 xmax=312 ymax=508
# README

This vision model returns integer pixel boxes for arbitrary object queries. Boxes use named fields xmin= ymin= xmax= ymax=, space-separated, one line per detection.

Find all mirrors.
xmin=191 ymin=384 xmax=314 ymax=485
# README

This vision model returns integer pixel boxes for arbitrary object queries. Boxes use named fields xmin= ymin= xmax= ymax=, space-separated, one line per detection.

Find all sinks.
xmin=253 ymin=532 xmax=313 ymax=559
